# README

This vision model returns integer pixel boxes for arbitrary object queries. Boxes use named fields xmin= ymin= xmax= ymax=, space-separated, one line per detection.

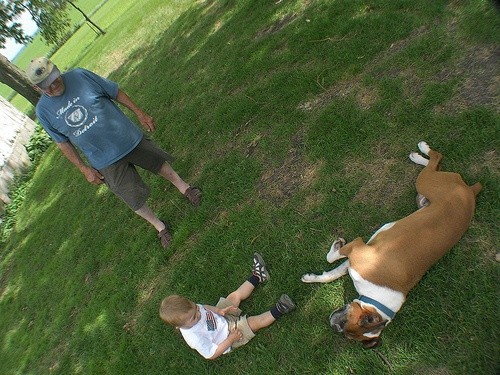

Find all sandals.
xmin=182 ymin=186 xmax=202 ymax=205
xmin=156 ymin=221 xmax=172 ymax=247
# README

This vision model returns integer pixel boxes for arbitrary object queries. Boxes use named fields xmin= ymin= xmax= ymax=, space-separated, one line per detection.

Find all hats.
xmin=27 ymin=57 xmax=61 ymax=89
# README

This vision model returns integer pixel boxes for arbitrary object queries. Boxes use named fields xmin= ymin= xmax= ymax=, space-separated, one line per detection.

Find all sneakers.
xmin=278 ymin=296 xmax=293 ymax=313
xmin=253 ymin=254 xmax=269 ymax=283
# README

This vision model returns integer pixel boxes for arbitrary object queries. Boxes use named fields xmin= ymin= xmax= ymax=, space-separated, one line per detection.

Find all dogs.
xmin=301 ymin=140 xmax=482 ymax=350
